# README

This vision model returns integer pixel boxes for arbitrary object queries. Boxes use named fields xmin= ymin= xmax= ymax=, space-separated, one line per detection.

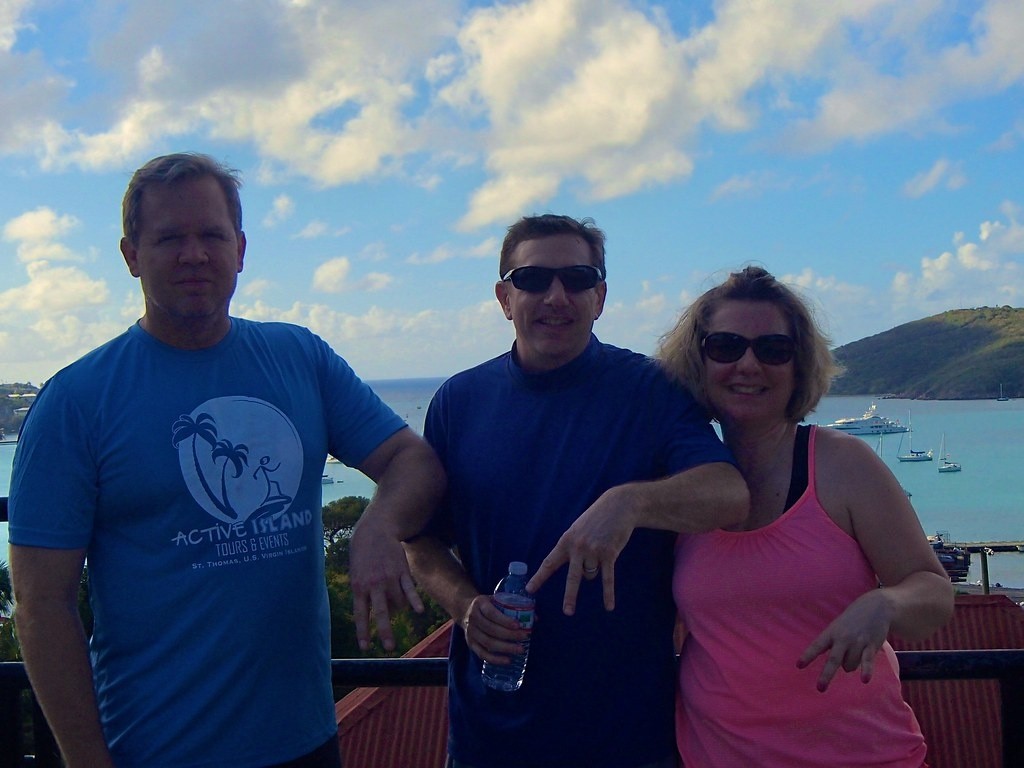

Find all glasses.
xmin=697 ymin=330 xmax=802 ymax=367
xmin=502 ymin=265 xmax=606 ymax=294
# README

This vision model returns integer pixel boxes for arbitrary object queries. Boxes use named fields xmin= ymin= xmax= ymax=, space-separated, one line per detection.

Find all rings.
xmin=585 ymin=568 xmax=597 ymax=572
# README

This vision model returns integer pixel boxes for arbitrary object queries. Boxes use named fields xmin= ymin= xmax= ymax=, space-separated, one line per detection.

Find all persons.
xmin=8 ymin=152 xmax=444 ymax=768
xmin=660 ymin=267 xmax=954 ymax=768
xmin=401 ymin=214 xmax=750 ymax=768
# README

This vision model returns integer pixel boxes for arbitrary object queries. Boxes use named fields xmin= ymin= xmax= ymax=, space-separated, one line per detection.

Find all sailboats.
xmin=936 ymin=429 xmax=962 ymax=473
xmin=895 ymin=406 xmax=933 ymax=462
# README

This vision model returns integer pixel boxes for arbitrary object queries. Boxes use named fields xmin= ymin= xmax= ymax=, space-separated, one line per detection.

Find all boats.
xmin=826 ymin=401 xmax=913 ymax=433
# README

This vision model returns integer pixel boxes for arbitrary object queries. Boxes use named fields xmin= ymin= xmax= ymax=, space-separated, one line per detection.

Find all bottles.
xmin=480 ymin=562 xmax=536 ymax=692
